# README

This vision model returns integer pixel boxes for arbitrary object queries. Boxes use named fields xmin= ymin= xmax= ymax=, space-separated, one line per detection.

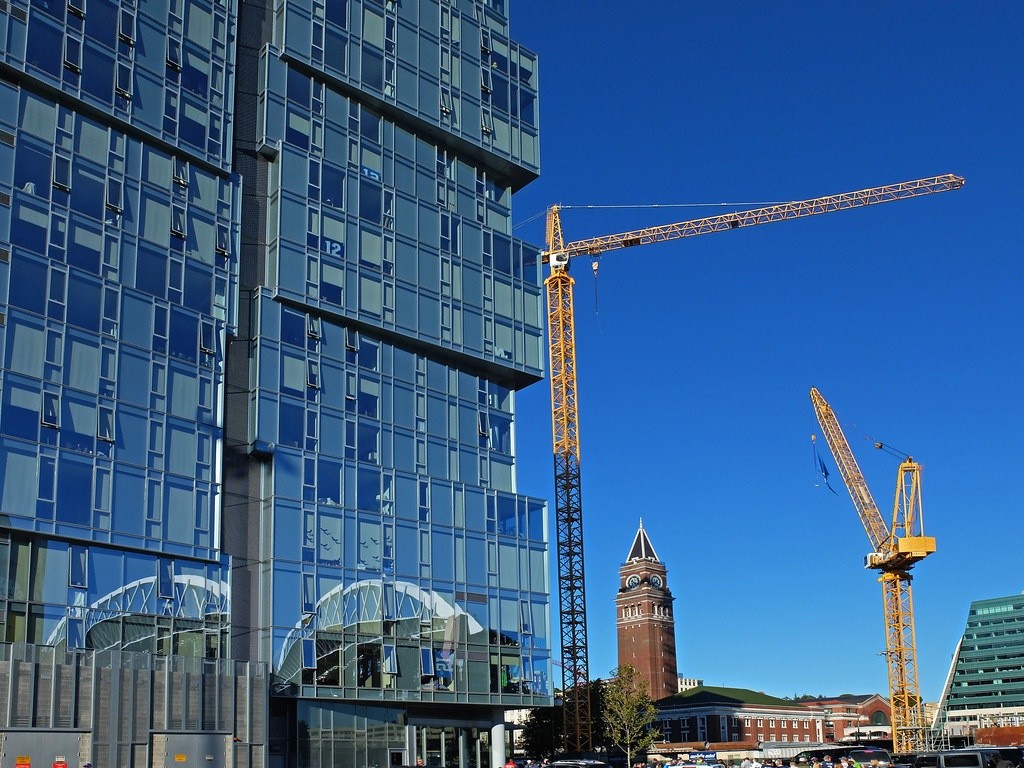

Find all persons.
xmin=632 ymin=757 xmax=740 ymax=768
xmin=504 ymin=758 xmax=551 ymax=768
xmin=741 ymin=755 xmax=895 ymax=768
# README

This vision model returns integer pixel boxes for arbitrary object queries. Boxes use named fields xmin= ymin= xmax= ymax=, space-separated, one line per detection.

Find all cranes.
xmin=808 ymin=385 xmax=936 ymax=755
xmin=540 ymin=173 xmax=966 ymax=758
